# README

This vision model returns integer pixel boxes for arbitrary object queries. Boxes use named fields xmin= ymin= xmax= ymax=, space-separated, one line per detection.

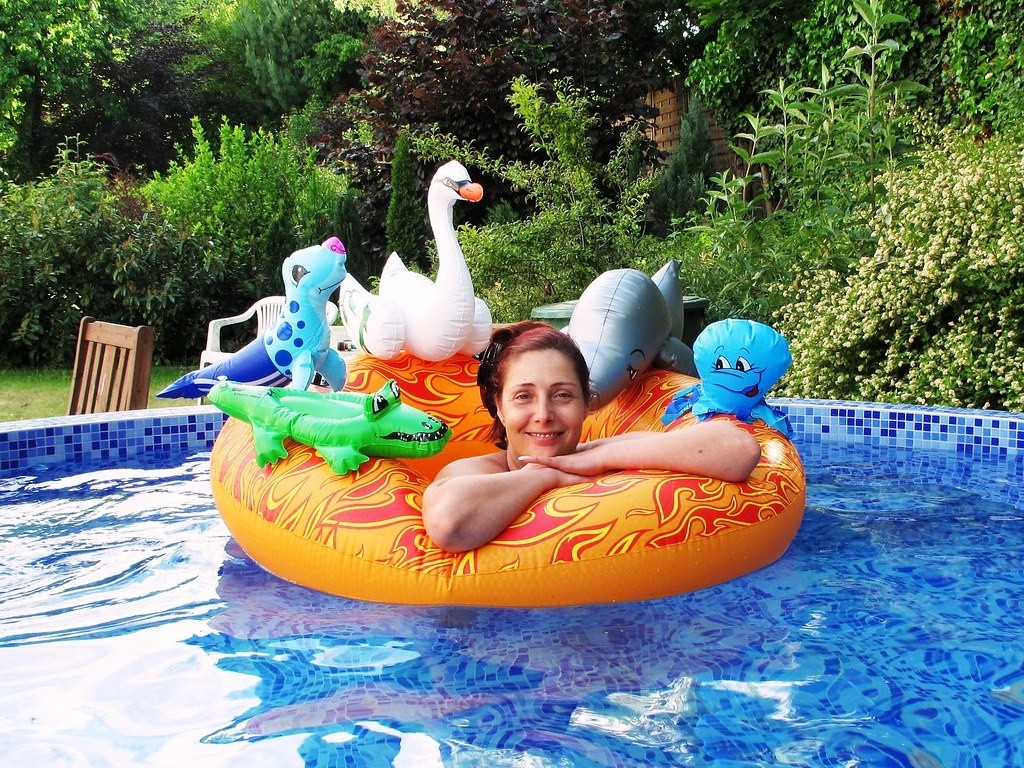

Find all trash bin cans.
xmin=531 ymin=299 xmax=578 ymax=329
xmin=681 ymin=295 xmax=711 ymax=350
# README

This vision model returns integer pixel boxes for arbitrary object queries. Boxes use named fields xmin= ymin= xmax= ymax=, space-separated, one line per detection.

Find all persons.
xmin=421 ymin=321 xmax=763 ymax=554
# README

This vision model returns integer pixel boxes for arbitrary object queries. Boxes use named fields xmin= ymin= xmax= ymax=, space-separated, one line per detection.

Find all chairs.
xmin=198 ymin=296 xmax=339 ymax=405
xmin=65 ymin=316 xmax=153 ymax=415
